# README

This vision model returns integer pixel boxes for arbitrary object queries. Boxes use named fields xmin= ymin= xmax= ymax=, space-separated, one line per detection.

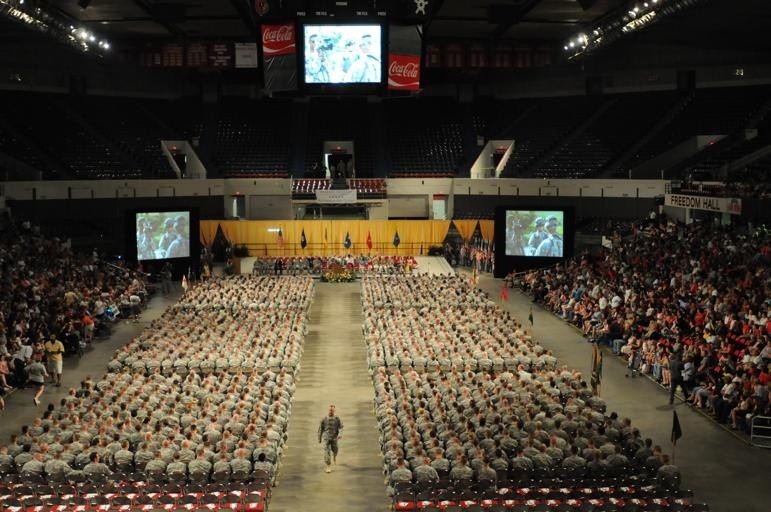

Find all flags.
xmin=367 ymin=233 xmax=372 ymax=249
xmin=301 ymin=229 xmax=307 ymax=248
xmin=344 ymin=233 xmax=351 ymax=248
xmin=393 ymin=232 xmax=401 ymax=247
xmin=278 ymin=229 xmax=284 ymax=247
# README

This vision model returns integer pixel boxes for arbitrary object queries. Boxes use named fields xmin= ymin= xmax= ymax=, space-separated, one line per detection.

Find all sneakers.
xmin=33 ymin=379 xmax=62 ymax=407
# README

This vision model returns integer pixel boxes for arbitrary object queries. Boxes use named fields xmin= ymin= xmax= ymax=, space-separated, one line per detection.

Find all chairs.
xmin=391 ymin=470 xmax=711 ymax=510
xmin=1 ymin=467 xmax=273 ymax=512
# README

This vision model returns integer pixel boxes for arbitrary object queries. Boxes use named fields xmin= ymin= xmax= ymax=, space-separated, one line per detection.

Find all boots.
xmin=326 ymin=455 xmax=337 ymax=473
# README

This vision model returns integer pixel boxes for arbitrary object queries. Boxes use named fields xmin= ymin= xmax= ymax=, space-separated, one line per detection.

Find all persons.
xmin=0 ymin=201 xmax=152 ymax=411
xmin=198 ymin=244 xmax=250 ymax=279
xmin=135 ymin=214 xmax=190 ymax=258
xmin=316 ymin=405 xmax=343 ymax=473
xmin=305 ymin=34 xmax=381 ymax=83
xmin=511 ymin=179 xmax=771 ymax=434
xmin=1 ymin=275 xmax=314 ymax=482
xmin=253 ymin=254 xmax=415 ymax=275
xmin=362 ymin=272 xmax=681 ymax=487
xmin=460 ymin=244 xmax=495 ymax=272
xmin=161 ymin=262 xmax=172 ymax=295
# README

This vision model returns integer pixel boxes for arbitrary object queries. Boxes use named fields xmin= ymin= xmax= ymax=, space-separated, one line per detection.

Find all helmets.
xmin=304 ymin=31 xmax=376 ymax=50
xmin=136 ymin=217 xmax=188 ymax=231
xmin=506 ymin=215 xmax=561 ymax=228
